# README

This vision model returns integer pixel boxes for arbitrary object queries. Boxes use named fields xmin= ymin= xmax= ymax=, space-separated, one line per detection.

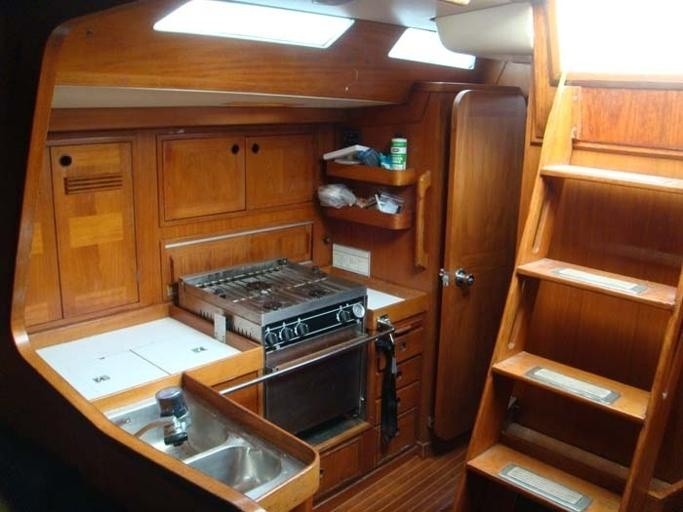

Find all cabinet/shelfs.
xmin=23 ymin=136 xmax=141 ymax=327
xmin=161 ymin=136 xmax=315 ymax=224
xmin=373 ymin=312 xmax=419 ymax=458
xmin=321 ymin=160 xmax=417 ymax=231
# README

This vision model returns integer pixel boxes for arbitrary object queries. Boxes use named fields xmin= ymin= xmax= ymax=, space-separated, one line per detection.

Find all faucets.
xmin=132 ymin=415 xmax=179 ymax=438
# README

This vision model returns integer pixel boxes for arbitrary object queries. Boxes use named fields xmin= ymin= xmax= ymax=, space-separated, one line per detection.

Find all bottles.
xmin=391 ymin=135 xmax=408 ymax=170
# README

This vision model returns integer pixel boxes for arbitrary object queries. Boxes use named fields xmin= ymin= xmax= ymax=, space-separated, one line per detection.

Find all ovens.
xmin=262 ymin=322 xmax=369 ymax=440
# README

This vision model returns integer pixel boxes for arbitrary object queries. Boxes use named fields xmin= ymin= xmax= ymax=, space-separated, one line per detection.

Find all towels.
xmin=382 ymin=340 xmax=397 ymax=457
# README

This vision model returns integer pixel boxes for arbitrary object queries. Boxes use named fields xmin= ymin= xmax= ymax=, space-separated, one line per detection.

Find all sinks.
xmin=102 ymin=389 xmax=229 ymax=460
xmin=187 ymin=443 xmax=306 ymax=503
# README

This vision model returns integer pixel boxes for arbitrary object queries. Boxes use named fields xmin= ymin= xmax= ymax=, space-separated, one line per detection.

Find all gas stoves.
xmin=183 ymin=257 xmax=368 ymax=347
